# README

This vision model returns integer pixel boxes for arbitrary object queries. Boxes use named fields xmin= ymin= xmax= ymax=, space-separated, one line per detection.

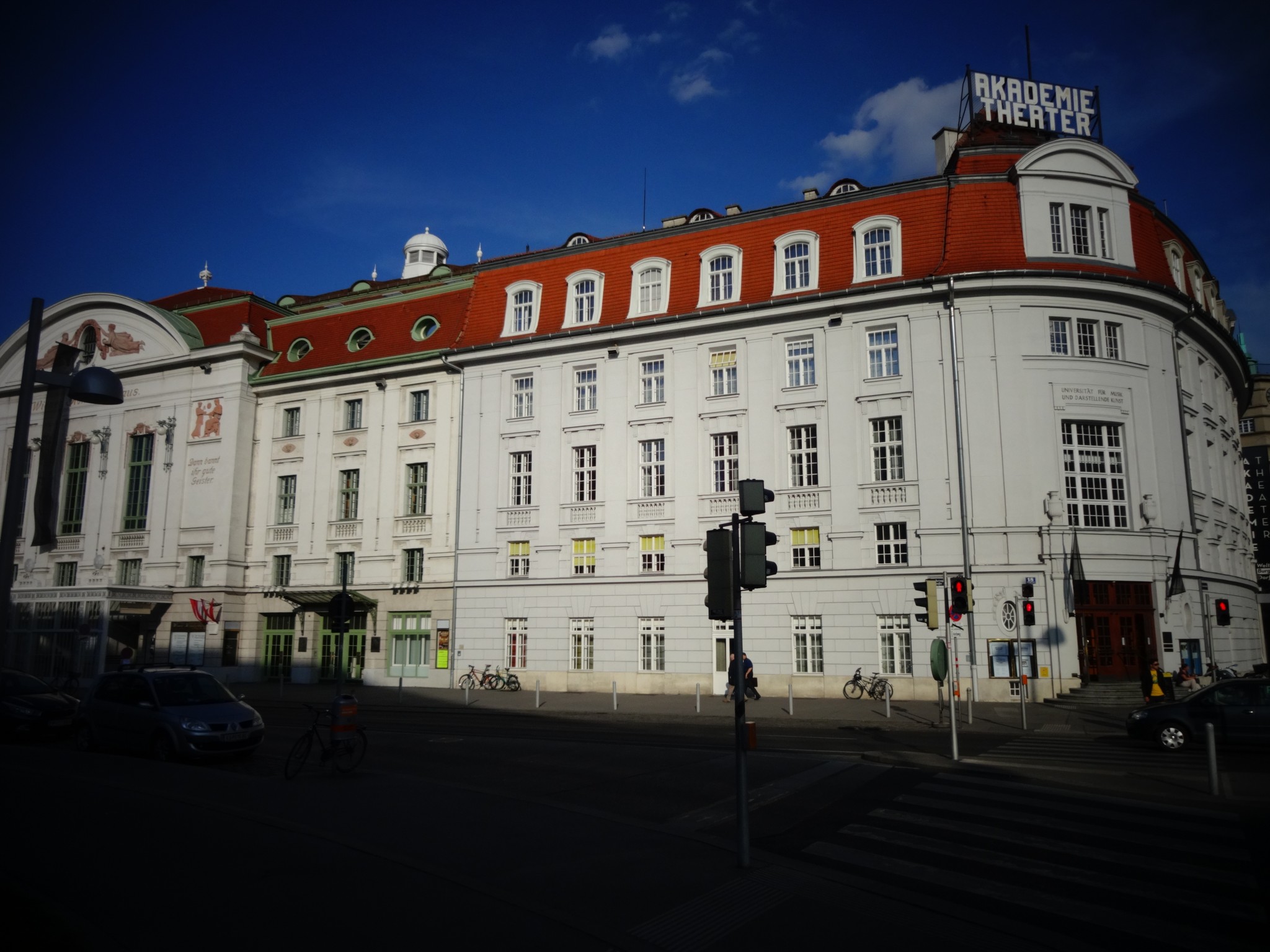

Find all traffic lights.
xmin=741 ymin=521 xmax=778 ymax=590
xmin=1021 ymin=583 xmax=1034 ymax=597
xmin=1215 ymin=599 xmax=1230 ymax=626
xmin=739 ymin=478 xmax=775 ymax=517
xmin=1023 ymin=600 xmax=1035 ymax=626
xmin=913 ymin=578 xmax=939 ymax=631
xmin=950 ymin=577 xmax=969 ymax=614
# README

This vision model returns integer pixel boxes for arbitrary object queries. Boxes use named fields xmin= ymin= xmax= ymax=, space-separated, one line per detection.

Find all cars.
xmin=80 ymin=662 xmax=266 ymax=765
xmin=0 ymin=669 xmax=83 ymax=743
xmin=1126 ymin=677 xmax=1270 ymax=768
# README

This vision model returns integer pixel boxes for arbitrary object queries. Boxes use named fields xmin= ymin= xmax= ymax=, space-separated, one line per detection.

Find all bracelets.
xmin=745 ymin=671 xmax=749 ymax=675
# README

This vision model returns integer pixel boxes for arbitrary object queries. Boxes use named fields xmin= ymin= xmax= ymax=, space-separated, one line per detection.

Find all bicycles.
xmin=49 ymin=670 xmax=82 ymax=693
xmin=284 ymin=701 xmax=368 ymax=780
xmin=458 ymin=665 xmax=498 ymax=691
xmin=489 ymin=666 xmax=520 ymax=691
xmin=843 ymin=667 xmax=894 ymax=702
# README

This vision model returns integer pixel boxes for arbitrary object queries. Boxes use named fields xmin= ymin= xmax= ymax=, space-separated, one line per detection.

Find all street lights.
xmin=0 ymin=297 xmax=125 ymax=669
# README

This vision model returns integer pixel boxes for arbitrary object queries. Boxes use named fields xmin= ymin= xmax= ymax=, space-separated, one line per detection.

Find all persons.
xmin=1175 ymin=663 xmax=1201 ymax=691
xmin=1141 ymin=657 xmax=1167 ymax=706
xmin=97 ymin=323 xmax=146 ymax=357
xmin=191 ymin=399 xmax=223 ymax=439
xmin=35 ymin=329 xmax=80 ymax=371
xmin=723 ymin=653 xmax=761 ymax=702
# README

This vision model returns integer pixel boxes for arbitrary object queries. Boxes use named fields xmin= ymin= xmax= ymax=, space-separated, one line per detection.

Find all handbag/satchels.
xmin=854 ymin=670 xmax=862 ymax=680
xmin=745 ymin=677 xmax=758 ymax=687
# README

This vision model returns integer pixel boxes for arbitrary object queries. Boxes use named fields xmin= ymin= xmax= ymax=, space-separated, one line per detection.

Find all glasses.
xmin=1153 ymin=663 xmax=1159 ymax=666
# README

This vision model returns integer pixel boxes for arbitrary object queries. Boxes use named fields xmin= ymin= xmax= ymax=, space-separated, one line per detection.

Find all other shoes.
xmin=744 ymin=699 xmax=748 ymax=702
xmin=722 ymin=699 xmax=730 ymax=702
xmin=754 ymin=695 xmax=761 ymax=700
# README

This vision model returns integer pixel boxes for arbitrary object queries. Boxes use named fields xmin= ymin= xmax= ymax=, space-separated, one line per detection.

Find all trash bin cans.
xmin=328 ymin=693 xmax=358 ymax=754
